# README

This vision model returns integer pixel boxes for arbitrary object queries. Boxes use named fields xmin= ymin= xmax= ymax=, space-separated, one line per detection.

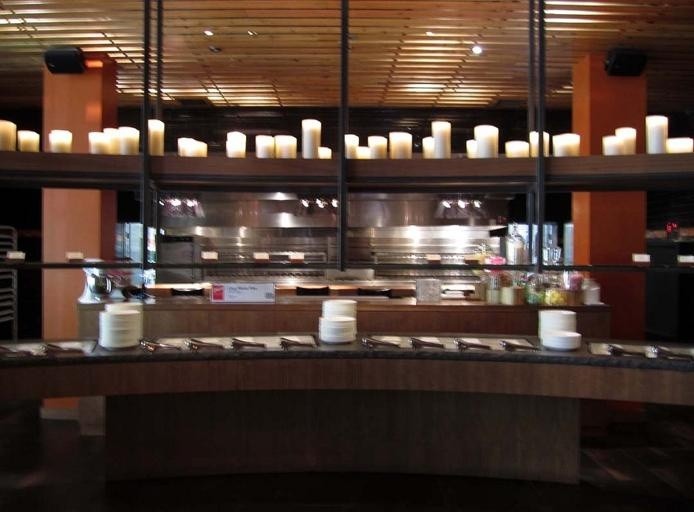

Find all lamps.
xmin=87 ymin=127 xmax=140 ymax=154
xmin=666 ymin=137 xmax=693 ymax=153
xmin=552 ymin=134 xmax=581 ymax=155
xmin=17 ymin=130 xmax=40 ymax=152
xmin=529 ymin=131 xmax=539 ymax=156
xmin=646 ymin=116 xmax=669 ymax=154
xmin=255 ymin=134 xmax=297 ymax=157
xmin=297 ymin=198 xmax=340 ymax=211
xmin=317 ymin=146 xmax=332 ymax=157
xmin=147 ymin=119 xmax=166 ymax=156
xmin=505 ymin=141 xmax=530 ymax=156
xmin=344 ymin=134 xmax=387 ymax=156
xmin=302 ymin=119 xmax=321 ymax=157
xmin=1 ymin=121 xmax=17 ymax=151
xmin=389 ymin=131 xmax=412 ymax=157
xmin=466 ymin=126 xmax=498 ymax=157
xmin=177 ymin=137 xmax=208 ymax=156
xmin=423 ymin=121 xmax=452 ymax=156
xmin=543 ymin=131 xmax=549 ymax=156
xmin=602 ymin=127 xmax=638 ymax=155
xmin=226 ymin=131 xmax=247 ymax=158
xmin=50 ymin=129 xmax=73 ymax=153
xmin=442 ymin=197 xmax=484 ymax=210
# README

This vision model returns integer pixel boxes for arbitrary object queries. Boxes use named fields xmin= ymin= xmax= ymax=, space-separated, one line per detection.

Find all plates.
xmin=318 ymin=298 xmax=358 ymax=345
xmin=98 ymin=301 xmax=144 ymax=351
xmin=538 ymin=309 xmax=583 ymax=351
